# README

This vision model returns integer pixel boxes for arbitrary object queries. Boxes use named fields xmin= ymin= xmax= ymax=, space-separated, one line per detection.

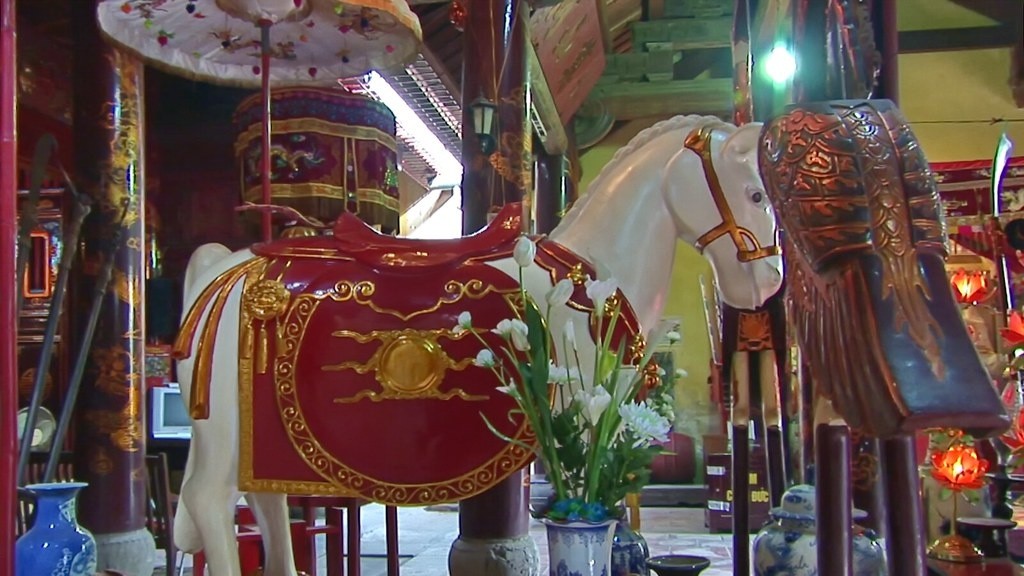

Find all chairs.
xmin=19 ymin=451 xmax=76 ymax=536
xmin=143 ymin=453 xmax=181 ymax=575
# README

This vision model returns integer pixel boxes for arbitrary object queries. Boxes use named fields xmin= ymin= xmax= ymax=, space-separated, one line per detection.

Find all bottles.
xmin=644 ymin=553 xmax=711 ymax=576
xmin=13 ymin=482 xmax=97 ymax=576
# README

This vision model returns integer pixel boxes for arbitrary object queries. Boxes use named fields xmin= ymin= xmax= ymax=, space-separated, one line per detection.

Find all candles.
xmin=732 ymin=424 xmax=749 ymax=576
xmin=878 ymin=435 xmax=928 ymax=576
xmin=816 ymin=423 xmax=854 ymax=576
xmin=767 ymin=424 xmax=785 ymax=507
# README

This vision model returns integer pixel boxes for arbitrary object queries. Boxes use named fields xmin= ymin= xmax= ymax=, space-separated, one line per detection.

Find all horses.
xmin=175 ymin=114 xmax=786 ymax=576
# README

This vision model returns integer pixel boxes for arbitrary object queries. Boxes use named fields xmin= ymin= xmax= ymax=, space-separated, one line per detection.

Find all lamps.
xmin=470 ymin=96 xmax=498 ymax=157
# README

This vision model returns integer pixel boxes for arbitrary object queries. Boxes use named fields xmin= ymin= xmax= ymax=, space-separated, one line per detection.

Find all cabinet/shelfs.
xmin=147 ymin=438 xmax=190 ymax=516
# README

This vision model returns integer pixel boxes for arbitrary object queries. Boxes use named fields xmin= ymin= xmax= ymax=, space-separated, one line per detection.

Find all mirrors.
xmin=23 ymin=231 xmax=50 ymax=298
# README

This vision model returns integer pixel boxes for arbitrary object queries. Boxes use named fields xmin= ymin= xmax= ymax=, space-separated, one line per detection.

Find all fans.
xmin=17 ymin=407 xmax=56 ymax=451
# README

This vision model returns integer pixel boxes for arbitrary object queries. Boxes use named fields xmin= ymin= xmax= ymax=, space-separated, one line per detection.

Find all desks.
xmin=957 ymin=517 xmax=1018 ymax=557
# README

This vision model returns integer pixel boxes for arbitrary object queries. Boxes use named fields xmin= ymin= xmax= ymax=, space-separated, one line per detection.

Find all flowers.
xmin=458 ymin=235 xmax=687 ymax=524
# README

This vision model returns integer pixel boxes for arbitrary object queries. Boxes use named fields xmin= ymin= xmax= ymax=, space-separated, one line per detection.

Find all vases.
xmin=611 ymin=505 xmax=651 ymax=576
xmin=645 ymin=554 xmax=710 ymax=576
xmin=14 ymin=482 xmax=97 ymax=575
xmin=539 ymin=518 xmax=618 ymax=576
